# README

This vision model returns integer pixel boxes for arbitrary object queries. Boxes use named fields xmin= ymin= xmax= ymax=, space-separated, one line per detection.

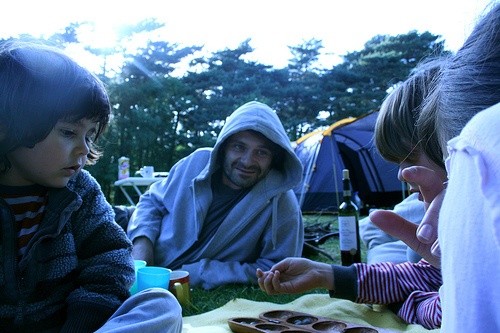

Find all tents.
xmin=291 ymin=110 xmax=412 ymax=216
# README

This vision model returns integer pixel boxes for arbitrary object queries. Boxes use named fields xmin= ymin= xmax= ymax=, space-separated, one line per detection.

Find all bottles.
xmin=338 ymin=168 xmax=362 ymax=267
xmin=118 ymin=157 xmax=129 ymax=180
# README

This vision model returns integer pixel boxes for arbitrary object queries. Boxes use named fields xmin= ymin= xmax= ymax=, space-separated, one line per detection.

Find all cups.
xmin=143 ymin=166 xmax=154 ymax=178
xmin=168 ymin=271 xmax=190 ymax=305
xmin=137 ymin=267 xmax=172 ymax=292
xmin=128 ymin=260 xmax=147 ymax=296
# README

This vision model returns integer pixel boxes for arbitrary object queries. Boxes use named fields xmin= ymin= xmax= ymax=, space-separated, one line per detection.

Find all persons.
xmin=0 ymin=42 xmax=182 ymax=333
xmin=255 ymin=8 xmax=500 ymax=333
xmin=113 ymin=101 xmax=305 ymax=293
